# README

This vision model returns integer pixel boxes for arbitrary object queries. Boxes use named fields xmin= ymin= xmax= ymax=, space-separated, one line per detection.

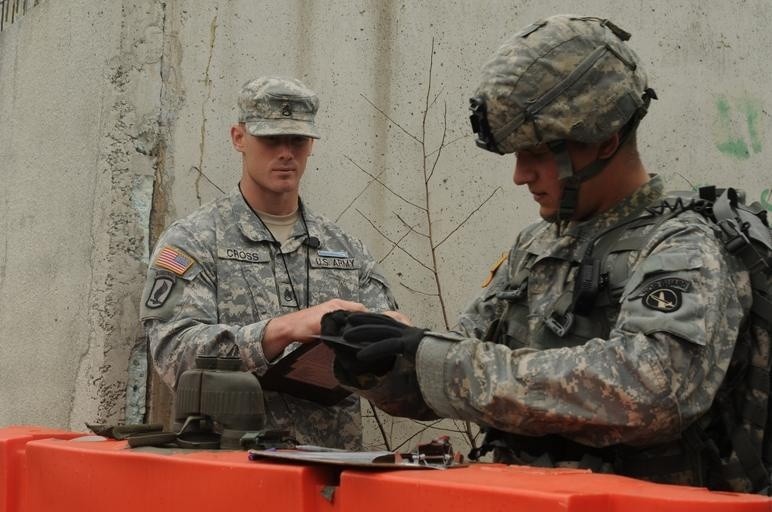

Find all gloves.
xmin=320 ymin=308 xmax=431 ymax=390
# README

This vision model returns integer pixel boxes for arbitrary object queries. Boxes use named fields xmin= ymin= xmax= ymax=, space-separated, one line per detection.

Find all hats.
xmin=237 ymin=75 xmax=322 ymax=141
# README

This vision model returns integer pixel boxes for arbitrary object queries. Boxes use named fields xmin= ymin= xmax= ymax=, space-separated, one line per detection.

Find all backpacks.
xmin=489 ymin=179 xmax=771 ymax=497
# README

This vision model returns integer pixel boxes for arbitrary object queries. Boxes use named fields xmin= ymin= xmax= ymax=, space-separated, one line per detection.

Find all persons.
xmin=133 ymin=74 xmax=417 ymax=453
xmin=315 ymin=11 xmax=756 ymax=489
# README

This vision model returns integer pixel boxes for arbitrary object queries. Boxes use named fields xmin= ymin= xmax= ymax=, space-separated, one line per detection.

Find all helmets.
xmin=468 ymin=13 xmax=649 ymax=156
